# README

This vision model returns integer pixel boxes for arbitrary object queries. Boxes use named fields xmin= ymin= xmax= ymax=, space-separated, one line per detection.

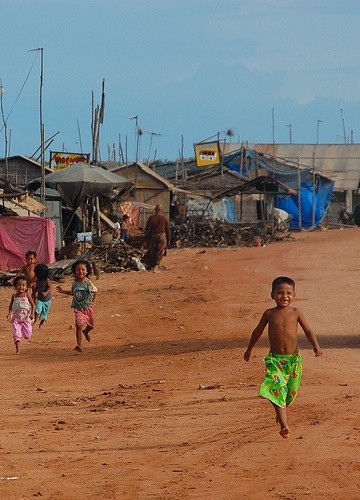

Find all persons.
xmin=6 ymin=250 xmax=52 ymax=355
xmin=121 ymin=215 xmax=129 ymax=242
xmin=244 ymin=277 xmax=322 ymax=438
xmin=145 ymin=203 xmax=171 ymax=273
xmin=56 ymin=260 xmax=98 ymax=352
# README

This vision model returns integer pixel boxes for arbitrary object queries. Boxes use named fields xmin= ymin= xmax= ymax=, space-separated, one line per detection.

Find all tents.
xmin=28 ymin=162 xmax=134 ymax=238
